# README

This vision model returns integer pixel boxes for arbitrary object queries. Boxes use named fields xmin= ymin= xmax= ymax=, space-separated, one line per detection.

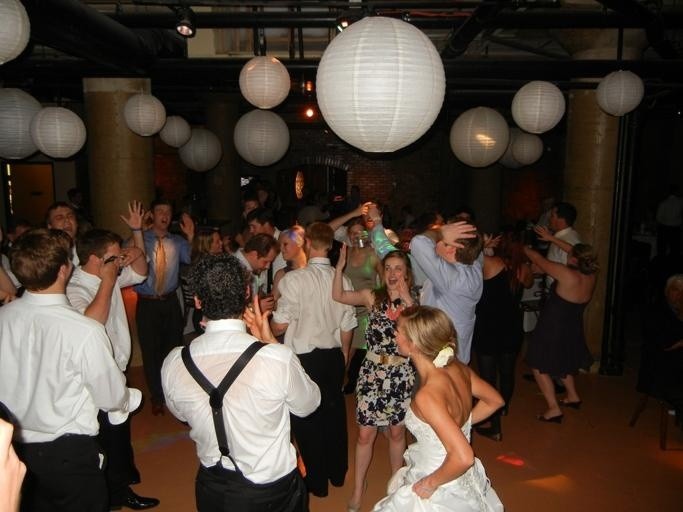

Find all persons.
xmin=1 ymin=200 xmax=160 ymax=511
xmin=161 ymin=189 xmax=598 ymax=511
xmin=642 ymin=273 xmax=683 ymax=354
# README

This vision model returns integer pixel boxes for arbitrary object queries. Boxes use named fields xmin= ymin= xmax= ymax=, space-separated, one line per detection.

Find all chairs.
xmin=628 ymin=324 xmax=683 ymax=450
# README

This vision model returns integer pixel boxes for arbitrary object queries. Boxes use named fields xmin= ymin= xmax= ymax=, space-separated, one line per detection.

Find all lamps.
xmin=124 ymin=2 xmax=316 ymax=174
xmin=0 ymin=0 xmax=86 ymax=163
xmin=315 ymin=14 xmax=447 ymax=158
xmin=450 ymin=68 xmax=644 ymax=173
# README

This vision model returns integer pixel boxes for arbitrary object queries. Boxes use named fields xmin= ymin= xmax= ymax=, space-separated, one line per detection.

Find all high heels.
xmin=558 ymin=398 xmax=582 ymax=409
xmin=536 ymin=411 xmax=564 ymax=423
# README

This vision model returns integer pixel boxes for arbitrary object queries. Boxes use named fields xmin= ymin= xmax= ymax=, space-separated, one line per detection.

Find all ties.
xmin=153 ymin=235 xmax=166 ymax=295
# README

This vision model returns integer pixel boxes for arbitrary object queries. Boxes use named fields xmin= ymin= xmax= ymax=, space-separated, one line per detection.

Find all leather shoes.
xmin=149 ymin=398 xmax=165 ymax=416
xmin=108 ymin=485 xmax=160 ymax=510
xmin=494 ymin=409 xmax=509 ymax=417
xmin=476 ymin=425 xmax=502 ymax=441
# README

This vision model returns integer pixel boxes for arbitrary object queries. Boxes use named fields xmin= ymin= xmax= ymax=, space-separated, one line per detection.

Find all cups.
xmin=355 ymin=230 xmax=370 ymax=249
